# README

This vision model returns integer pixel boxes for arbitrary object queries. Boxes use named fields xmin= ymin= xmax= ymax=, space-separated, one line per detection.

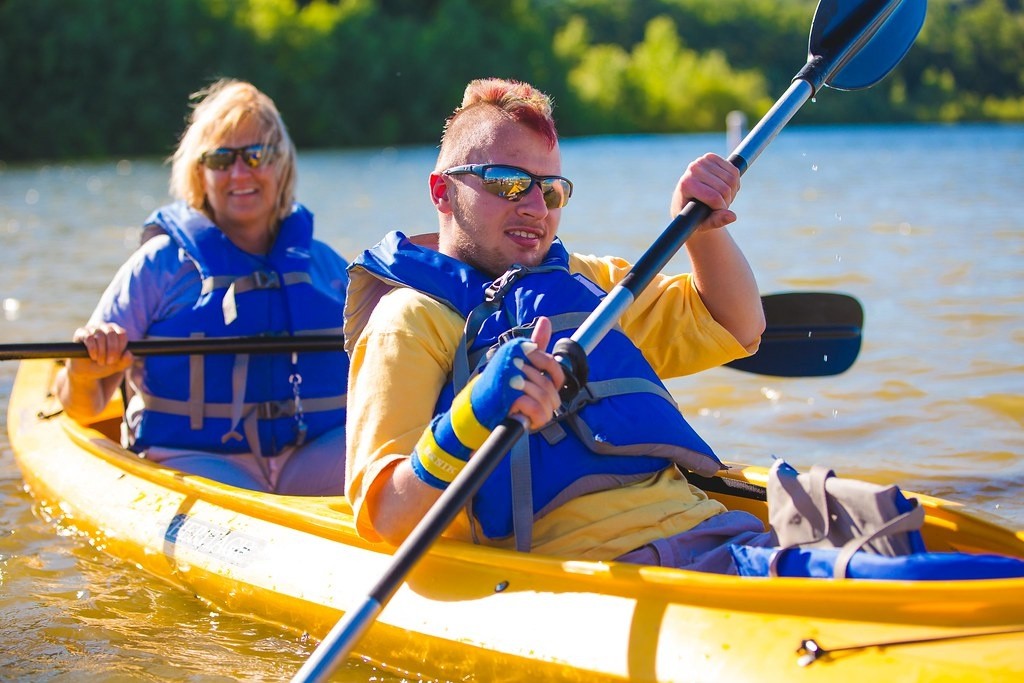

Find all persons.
xmin=343 ymin=75 xmax=778 ymax=575
xmin=51 ymin=81 xmax=353 ymax=498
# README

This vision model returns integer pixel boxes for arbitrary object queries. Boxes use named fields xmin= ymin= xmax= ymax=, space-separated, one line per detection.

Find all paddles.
xmin=0 ymin=291 xmax=866 ymax=378
xmin=291 ymin=0 xmax=930 ymax=683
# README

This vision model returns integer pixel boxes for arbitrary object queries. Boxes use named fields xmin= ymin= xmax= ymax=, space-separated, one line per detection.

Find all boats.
xmin=8 ymin=358 xmax=1024 ymax=683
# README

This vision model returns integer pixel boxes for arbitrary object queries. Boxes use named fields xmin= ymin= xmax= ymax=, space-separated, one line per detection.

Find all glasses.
xmin=441 ymin=163 xmax=574 ymax=210
xmin=198 ymin=142 xmax=279 ymax=171
